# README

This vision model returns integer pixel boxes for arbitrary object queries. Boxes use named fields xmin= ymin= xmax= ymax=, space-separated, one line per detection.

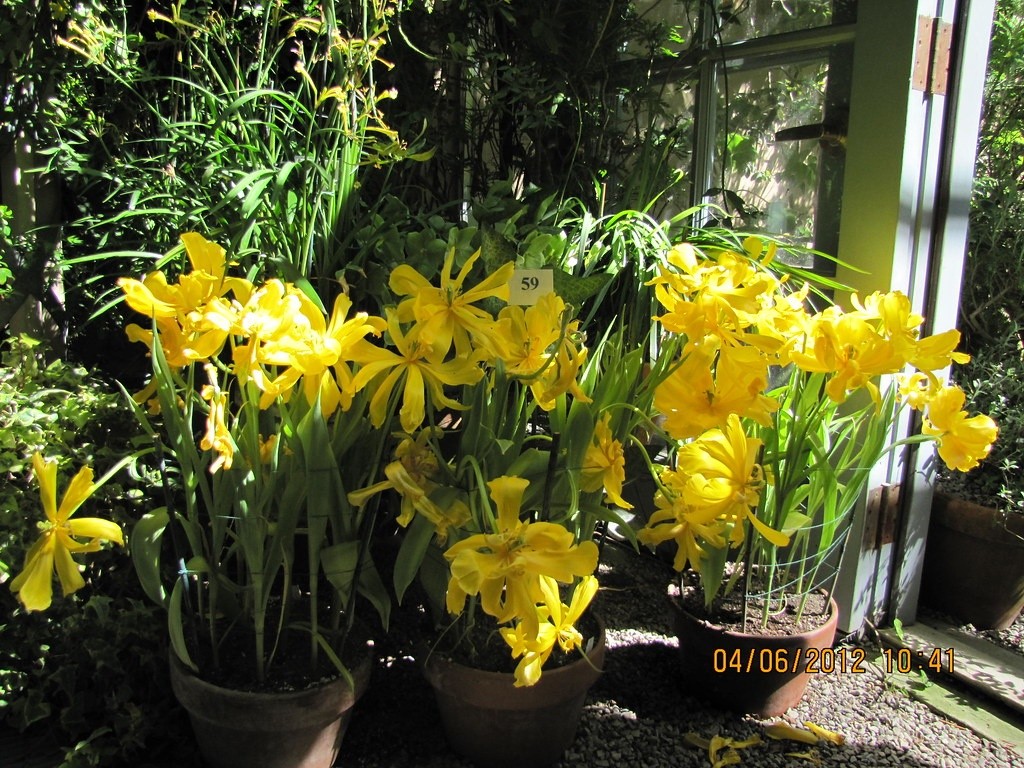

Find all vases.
xmin=666 ymin=561 xmax=838 ymax=717
xmin=167 ymin=602 xmax=370 ymax=768
xmin=423 ymin=605 xmax=605 ymax=768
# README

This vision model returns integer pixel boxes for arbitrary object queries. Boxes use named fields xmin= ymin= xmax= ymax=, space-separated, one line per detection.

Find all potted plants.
xmin=915 ymin=308 xmax=1024 ymax=635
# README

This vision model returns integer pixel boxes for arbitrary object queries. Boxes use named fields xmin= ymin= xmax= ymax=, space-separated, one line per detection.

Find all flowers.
xmin=7 ymin=232 xmax=1001 ymax=689
